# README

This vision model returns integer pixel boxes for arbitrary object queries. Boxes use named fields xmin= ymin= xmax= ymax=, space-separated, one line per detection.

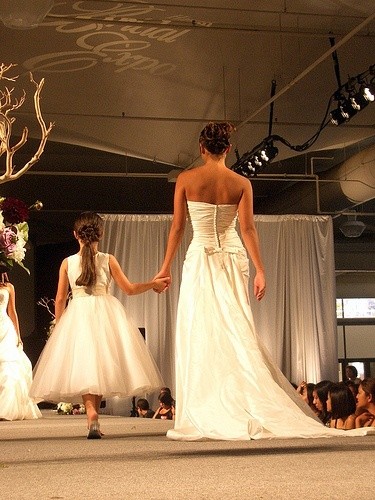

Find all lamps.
xmin=328 ymin=36 xmax=375 ymax=127
xmin=228 ymin=76 xmax=278 ymax=180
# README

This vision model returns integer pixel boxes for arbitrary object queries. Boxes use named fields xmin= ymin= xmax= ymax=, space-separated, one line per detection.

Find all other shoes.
xmin=86 ymin=423 xmax=102 ymax=439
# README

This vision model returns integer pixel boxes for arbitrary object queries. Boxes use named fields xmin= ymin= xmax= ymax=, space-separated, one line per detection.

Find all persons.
xmin=0 ymin=265 xmax=375 ymax=433
xmin=154 ymin=122 xmax=375 ymax=440
xmin=31 ymin=211 xmax=170 ymax=439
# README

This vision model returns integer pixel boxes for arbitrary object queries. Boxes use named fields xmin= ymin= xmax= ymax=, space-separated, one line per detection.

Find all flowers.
xmin=0 ymin=197 xmax=31 ymax=274
xmin=57 ymin=401 xmax=85 ymax=415
